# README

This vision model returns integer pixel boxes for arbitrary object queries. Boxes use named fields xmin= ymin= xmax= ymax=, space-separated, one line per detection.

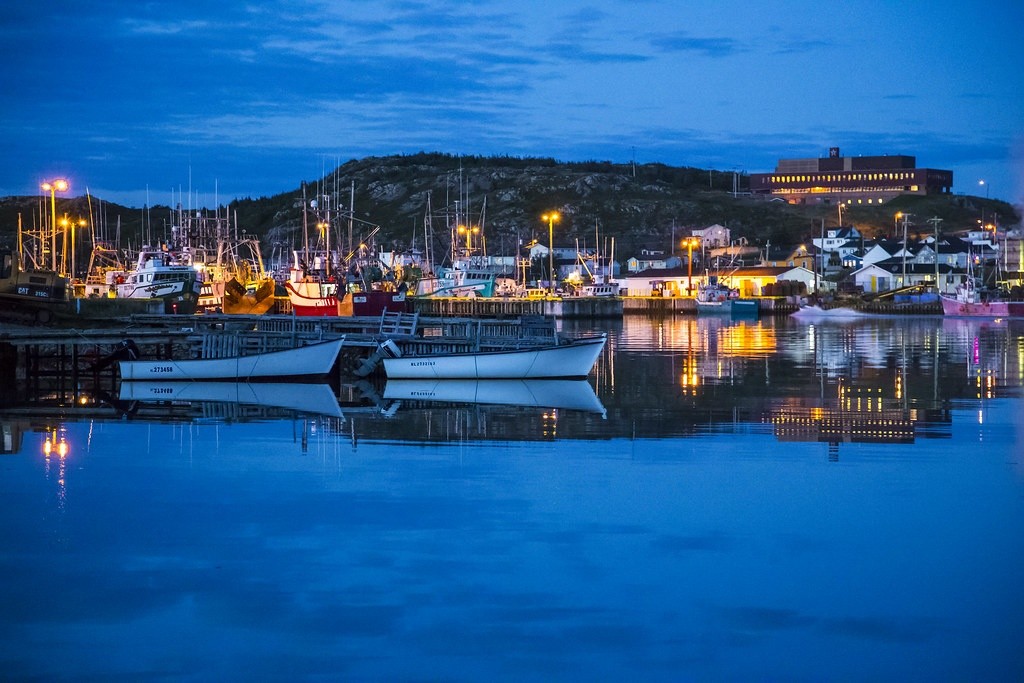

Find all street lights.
xmin=41 ymin=178 xmax=70 ymax=272
xmin=58 ymin=217 xmax=89 ymax=280
xmin=681 ymin=237 xmax=701 ymax=297
xmin=541 ymin=211 xmax=560 ymax=292
xmin=317 ymin=223 xmax=329 ymax=246
xmin=836 ymin=202 xmax=846 ymax=226
xmin=457 ymin=225 xmax=482 ymax=259
xmin=893 ymin=211 xmax=903 ymax=237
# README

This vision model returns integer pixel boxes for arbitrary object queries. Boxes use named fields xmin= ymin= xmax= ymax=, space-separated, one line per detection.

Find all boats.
xmin=84 ymin=375 xmax=348 ymax=424
xmin=693 ymin=256 xmax=761 ymax=317
xmin=936 ymin=239 xmax=1024 ymax=319
xmin=355 ymin=376 xmax=608 ymax=421
xmin=90 ymin=331 xmax=347 ymax=380
xmin=354 ymin=314 xmax=608 ymax=380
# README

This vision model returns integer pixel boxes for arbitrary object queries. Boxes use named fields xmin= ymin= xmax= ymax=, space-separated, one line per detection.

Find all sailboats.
xmin=1 ymin=150 xmax=624 ymax=318
